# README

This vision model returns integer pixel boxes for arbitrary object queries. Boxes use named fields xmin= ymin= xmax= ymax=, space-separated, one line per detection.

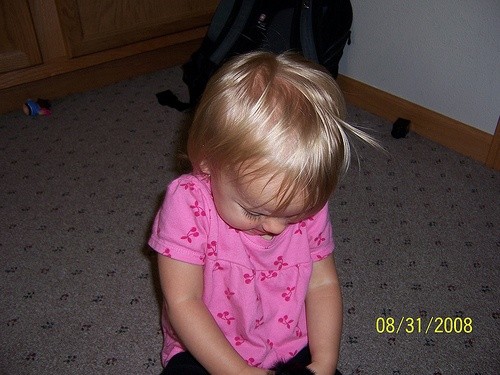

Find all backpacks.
xmin=156 ymin=0 xmax=353 ymax=112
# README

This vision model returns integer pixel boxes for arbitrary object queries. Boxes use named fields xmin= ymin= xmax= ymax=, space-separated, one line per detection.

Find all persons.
xmin=148 ymin=50 xmax=353 ymax=375
